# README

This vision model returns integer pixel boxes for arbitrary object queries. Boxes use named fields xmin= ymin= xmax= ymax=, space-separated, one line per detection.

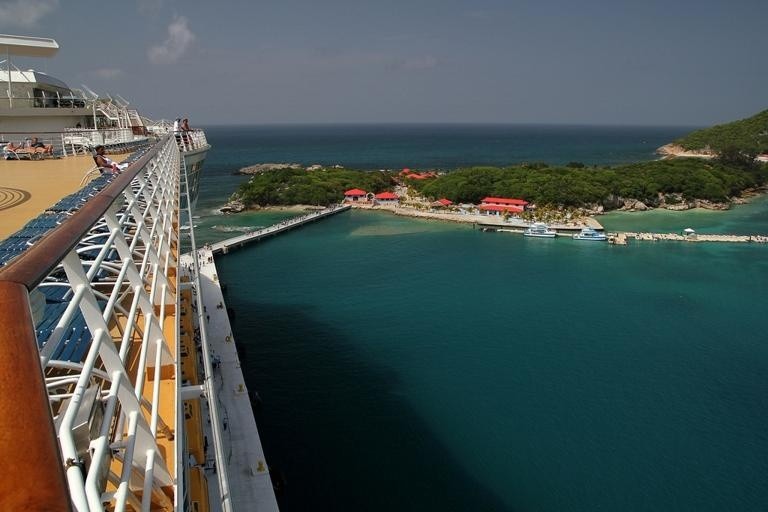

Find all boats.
xmin=524 ymin=222 xmax=556 ymax=238
xmin=573 ymin=227 xmax=606 ymax=241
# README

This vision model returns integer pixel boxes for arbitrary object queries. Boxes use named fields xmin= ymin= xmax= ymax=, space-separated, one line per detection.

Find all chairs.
xmin=1 ymin=148 xmax=167 ymax=367
xmin=5 ymin=141 xmax=56 ymax=161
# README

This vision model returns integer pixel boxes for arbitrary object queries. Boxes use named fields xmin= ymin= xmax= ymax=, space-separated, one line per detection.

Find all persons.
xmin=94 ymin=145 xmax=134 ymax=172
xmin=222 ymin=417 xmax=228 ymax=431
xmin=173 ymin=116 xmax=188 ymax=152
xmin=29 ymin=137 xmax=45 ymax=154
xmin=180 ymin=203 xmax=344 ymax=372
xmin=6 ymin=142 xmax=21 ymax=152
xmin=180 ymin=118 xmax=194 ymax=147
xmin=212 ymin=461 xmax=216 ymax=473
